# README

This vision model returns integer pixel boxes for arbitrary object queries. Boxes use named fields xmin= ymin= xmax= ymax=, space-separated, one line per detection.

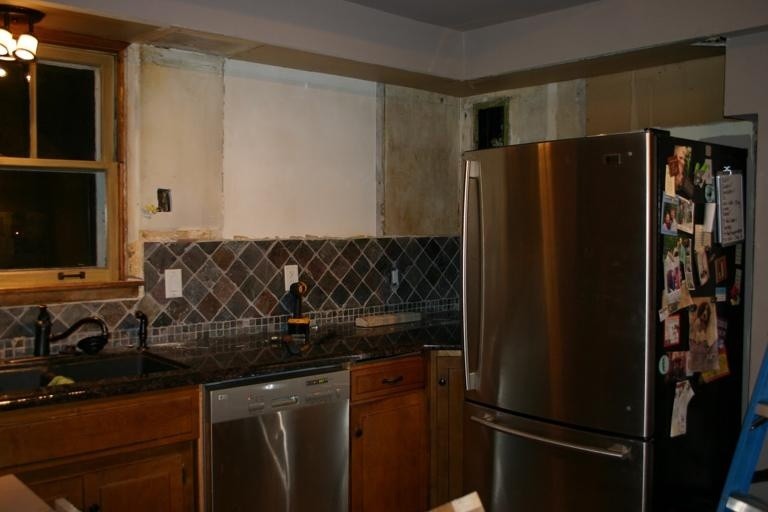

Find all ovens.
xmin=207 ymin=364 xmax=352 ymax=511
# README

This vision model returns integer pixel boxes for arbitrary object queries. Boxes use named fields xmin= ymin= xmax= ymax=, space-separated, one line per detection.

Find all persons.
xmin=675 ymin=145 xmax=693 ymax=195
xmin=662 ymin=211 xmax=670 ymax=232
xmin=668 ymin=207 xmax=679 ymax=233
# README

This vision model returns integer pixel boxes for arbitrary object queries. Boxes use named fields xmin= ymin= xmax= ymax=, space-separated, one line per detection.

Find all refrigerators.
xmin=458 ymin=128 xmax=747 ymax=512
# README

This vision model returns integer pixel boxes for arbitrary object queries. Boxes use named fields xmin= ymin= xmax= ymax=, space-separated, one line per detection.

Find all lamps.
xmin=0 ymin=3 xmax=45 ymax=62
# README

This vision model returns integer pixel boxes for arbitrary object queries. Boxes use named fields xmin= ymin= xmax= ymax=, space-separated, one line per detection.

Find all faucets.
xmin=49 ymin=314 xmax=110 ymax=354
xmin=134 ymin=309 xmax=148 ymax=349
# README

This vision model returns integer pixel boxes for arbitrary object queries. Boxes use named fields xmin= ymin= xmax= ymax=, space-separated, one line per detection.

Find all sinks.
xmin=0 ymin=363 xmax=61 ymax=395
xmin=53 ymin=349 xmax=193 ymax=388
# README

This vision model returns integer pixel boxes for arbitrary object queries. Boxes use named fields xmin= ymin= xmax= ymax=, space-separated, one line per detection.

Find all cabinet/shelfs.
xmin=0 ymin=386 xmax=199 ymax=511
xmin=347 ymin=350 xmax=465 ymax=512
